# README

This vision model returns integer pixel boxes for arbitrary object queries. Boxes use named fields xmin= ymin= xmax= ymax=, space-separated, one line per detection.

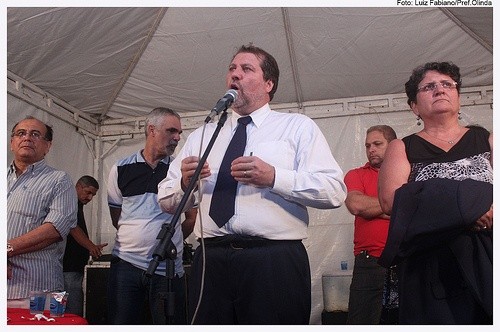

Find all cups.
xmin=30 ymin=290 xmax=46 ymax=315
xmin=49 ymin=291 xmax=69 ymax=317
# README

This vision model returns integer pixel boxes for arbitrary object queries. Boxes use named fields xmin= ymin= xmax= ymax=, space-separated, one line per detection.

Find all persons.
xmin=377 ymin=59 xmax=493 ymax=324
xmin=106 ymin=107 xmax=202 ymax=324
xmin=343 ymin=125 xmax=398 ymax=325
xmin=7 ymin=114 xmax=80 ymax=310
xmin=60 ymin=174 xmax=109 ymax=316
xmin=157 ymin=41 xmax=348 ymax=324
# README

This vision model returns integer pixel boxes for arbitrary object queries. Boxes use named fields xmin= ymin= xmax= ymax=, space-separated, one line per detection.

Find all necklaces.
xmin=422 ymin=125 xmax=463 ymax=144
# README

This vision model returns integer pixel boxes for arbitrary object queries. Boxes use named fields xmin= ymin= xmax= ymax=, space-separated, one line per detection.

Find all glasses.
xmin=414 ymin=82 xmax=458 ymax=97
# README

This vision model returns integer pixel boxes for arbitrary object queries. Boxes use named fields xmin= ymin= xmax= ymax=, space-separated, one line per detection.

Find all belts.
xmin=197 ymin=237 xmax=304 ymax=248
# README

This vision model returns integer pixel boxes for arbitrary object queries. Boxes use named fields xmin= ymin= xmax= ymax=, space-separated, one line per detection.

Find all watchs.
xmin=7 ymin=240 xmax=15 ymax=257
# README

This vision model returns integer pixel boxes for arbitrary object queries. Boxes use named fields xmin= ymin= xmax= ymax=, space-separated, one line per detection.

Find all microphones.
xmin=204 ymin=89 xmax=239 ymax=123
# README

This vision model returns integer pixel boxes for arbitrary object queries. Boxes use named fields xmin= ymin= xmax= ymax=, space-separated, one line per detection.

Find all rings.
xmin=480 ymin=223 xmax=487 ymax=230
xmin=243 ymin=170 xmax=247 ymax=176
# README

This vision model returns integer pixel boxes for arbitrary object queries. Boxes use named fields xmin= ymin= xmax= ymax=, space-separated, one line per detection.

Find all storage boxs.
xmin=83 ymin=265 xmax=112 ymax=325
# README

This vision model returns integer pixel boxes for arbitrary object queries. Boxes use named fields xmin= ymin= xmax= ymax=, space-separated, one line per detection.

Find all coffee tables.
xmin=7 ymin=307 xmax=88 ymax=325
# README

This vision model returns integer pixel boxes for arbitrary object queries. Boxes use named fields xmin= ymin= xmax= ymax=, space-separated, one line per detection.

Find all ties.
xmin=207 ymin=116 xmax=253 ymax=229
